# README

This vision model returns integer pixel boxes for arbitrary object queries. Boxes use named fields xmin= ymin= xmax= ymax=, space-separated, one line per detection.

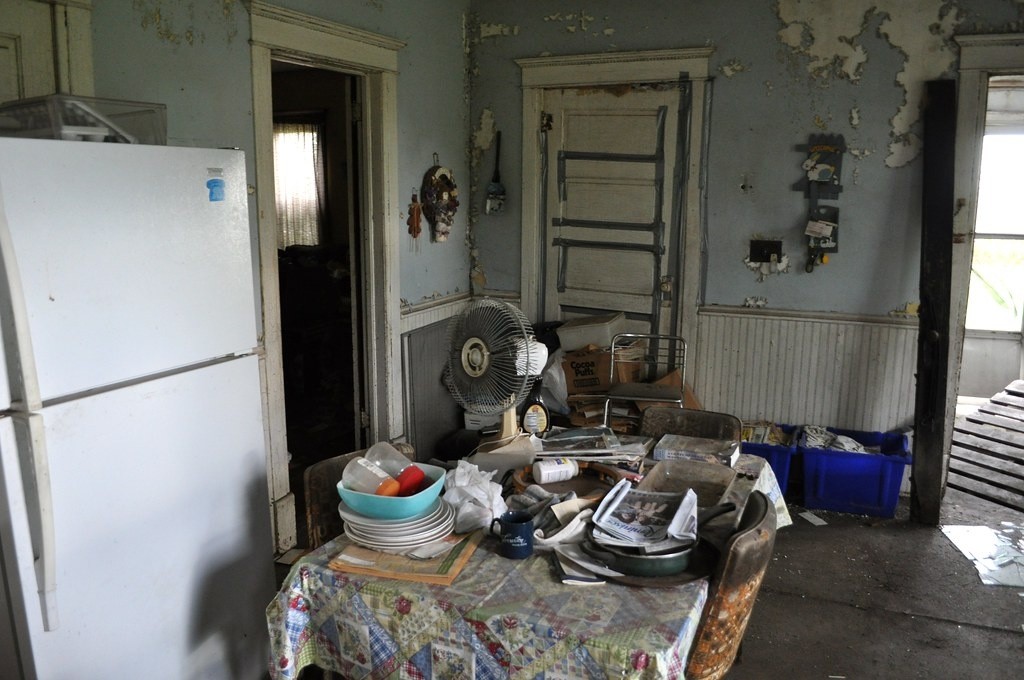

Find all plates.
xmin=338 ymin=500 xmax=456 ymax=549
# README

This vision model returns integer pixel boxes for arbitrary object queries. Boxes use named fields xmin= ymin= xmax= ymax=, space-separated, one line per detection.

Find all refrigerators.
xmin=0 ymin=136 xmax=284 ymax=680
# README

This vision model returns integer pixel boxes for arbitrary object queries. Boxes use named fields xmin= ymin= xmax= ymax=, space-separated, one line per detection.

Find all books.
xmin=529 ymin=426 xmax=743 ymax=468
xmin=590 ymin=476 xmax=698 ymax=555
xmin=551 ymin=547 xmax=607 ymax=585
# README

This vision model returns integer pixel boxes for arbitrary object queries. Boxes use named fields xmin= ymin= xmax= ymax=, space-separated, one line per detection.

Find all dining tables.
xmin=302 ymin=426 xmax=767 ymax=675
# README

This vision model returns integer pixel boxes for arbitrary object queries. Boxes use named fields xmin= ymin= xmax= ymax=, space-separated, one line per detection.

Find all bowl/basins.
xmin=336 ymin=461 xmax=446 ymax=519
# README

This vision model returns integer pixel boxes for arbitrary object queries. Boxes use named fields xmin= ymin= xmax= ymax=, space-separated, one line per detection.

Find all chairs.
xmin=685 ymin=490 xmax=778 ymax=680
xmin=603 ymin=333 xmax=688 ymax=427
xmin=303 ymin=443 xmax=422 ymax=552
xmin=636 ymin=407 xmax=743 ymax=447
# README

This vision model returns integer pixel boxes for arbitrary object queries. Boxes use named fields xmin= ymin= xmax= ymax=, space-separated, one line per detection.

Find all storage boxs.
xmin=799 ymin=424 xmax=913 ymax=518
xmin=1 ymin=92 xmax=167 ymax=145
xmin=739 ymin=419 xmax=799 ymax=498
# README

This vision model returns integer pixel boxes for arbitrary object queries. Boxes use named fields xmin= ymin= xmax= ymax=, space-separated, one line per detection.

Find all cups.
xmin=489 ymin=511 xmax=534 ymax=559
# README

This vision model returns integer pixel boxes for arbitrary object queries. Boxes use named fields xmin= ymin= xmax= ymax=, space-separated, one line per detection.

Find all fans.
xmin=443 ymin=296 xmax=548 ymax=457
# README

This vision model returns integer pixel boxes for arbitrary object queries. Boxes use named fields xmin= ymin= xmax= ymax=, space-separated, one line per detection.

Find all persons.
xmin=616 ymin=495 xmax=676 ymax=525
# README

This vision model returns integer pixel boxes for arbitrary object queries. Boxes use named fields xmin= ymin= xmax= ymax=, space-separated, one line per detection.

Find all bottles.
xmin=520 ymin=376 xmax=550 ymax=438
xmin=365 ymin=441 xmax=426 ymax=494
xmin=342 ymin=456 xmax=400 ymax=495
xmin=532 ymin=458 xmax=578 ymax=485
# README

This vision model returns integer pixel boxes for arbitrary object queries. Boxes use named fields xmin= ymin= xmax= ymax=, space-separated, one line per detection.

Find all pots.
xmin=580 ymin=501 xmax=737 ymax=576
